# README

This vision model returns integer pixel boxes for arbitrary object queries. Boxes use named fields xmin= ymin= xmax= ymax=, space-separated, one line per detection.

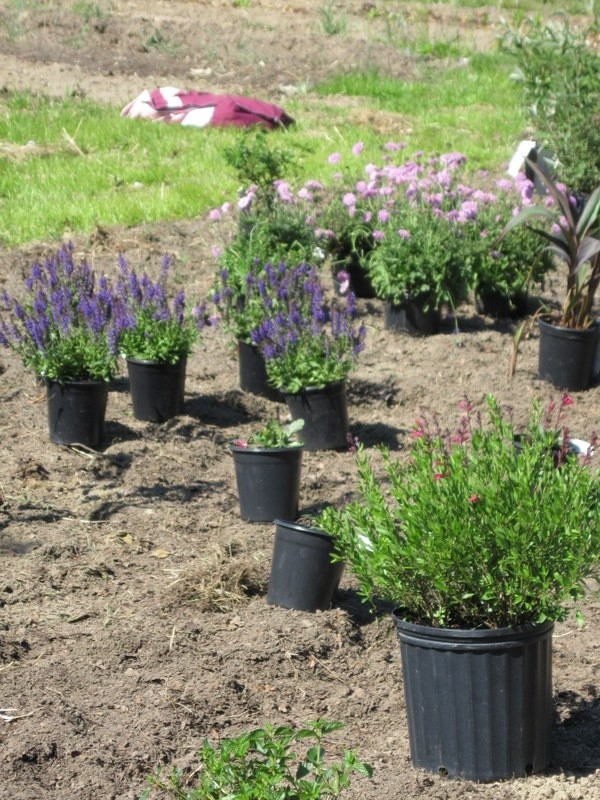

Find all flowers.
xmin=0 ymin=141 xmax=600 ymax=628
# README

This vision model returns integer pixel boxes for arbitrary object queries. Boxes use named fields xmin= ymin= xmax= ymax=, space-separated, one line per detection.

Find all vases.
xmin=268 ymin=519 xmax=346 ymax=610
xmin=238 ymin=336 xmax=286 ymax=401
xmin=331 ymin=251 xmax=378 ymax=298
xmin=277 ymin=380 xmax=350 ymax=452
xmin=384 ymin=292 xmax=442 ymax=336
xmin=230 ymin=444 xmax=301 ymax=520
xmin=45 ymin=377 xmax=109 ymax=447
xmin=124 ymin=352 xmax=186 ymax=422
xmin=390 ymin=608 xmax=558 ymax=780
xmin=476 ymin=281 xmax=528 ymax=318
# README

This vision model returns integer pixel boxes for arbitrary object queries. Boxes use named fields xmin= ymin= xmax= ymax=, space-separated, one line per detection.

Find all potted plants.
xmin=497 ymin=157 xmax=600 ymax=391
